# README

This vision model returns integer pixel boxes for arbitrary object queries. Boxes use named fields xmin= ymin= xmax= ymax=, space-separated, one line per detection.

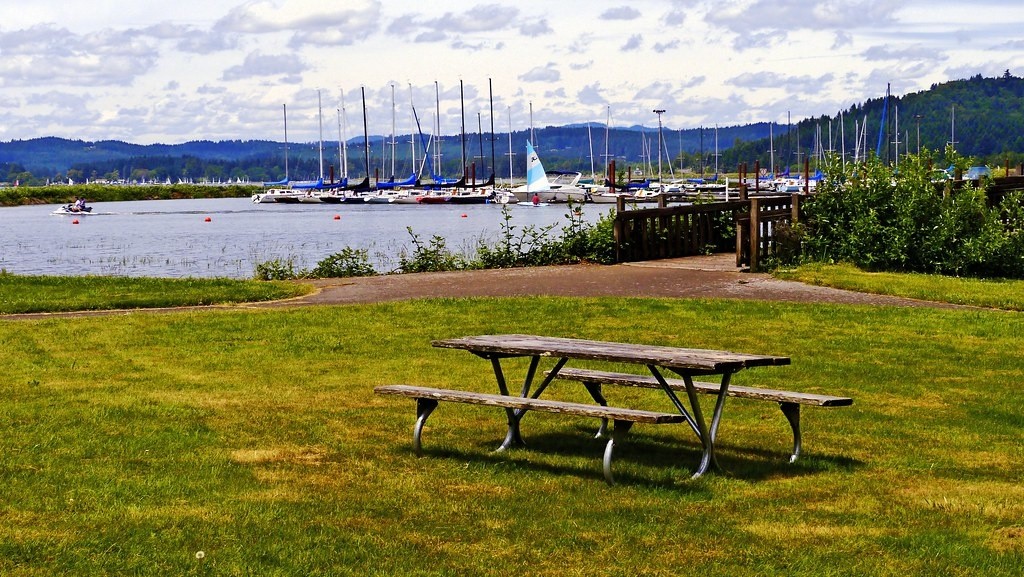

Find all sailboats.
xmin=251 ymin=78 xmax=973 ymax=203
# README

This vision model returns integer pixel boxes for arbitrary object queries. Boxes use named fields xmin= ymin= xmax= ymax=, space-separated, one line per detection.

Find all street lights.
xmin=654 ymin=110 xmax=665 ymax=195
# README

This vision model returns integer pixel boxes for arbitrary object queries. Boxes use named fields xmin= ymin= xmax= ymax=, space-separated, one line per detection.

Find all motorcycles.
xmin=57 ymin=204 xmax=92 ymax=215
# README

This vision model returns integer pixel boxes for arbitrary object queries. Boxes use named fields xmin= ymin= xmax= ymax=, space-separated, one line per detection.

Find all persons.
xmin=70 ymin=195 xmax=86 ymax=212
xmin=532 ymin=193 xmax=540 ymax=206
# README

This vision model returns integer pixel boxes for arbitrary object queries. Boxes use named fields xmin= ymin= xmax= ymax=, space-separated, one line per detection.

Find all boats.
xmin=510 ymin=169 xmax=588 ymax=202
xmin=485 ymin=182 xmax=520 ymax=204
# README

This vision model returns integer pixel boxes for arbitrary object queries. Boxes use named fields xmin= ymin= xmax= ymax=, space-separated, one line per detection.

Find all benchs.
xmin=544 ymin=366 xmax=854 ymax=466
xmin=373 ymin=383 xmax=687 ymax=491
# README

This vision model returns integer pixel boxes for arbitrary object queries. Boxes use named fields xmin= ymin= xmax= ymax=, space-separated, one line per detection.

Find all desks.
xmin=431 ymin=333 xmax=791 ymax=490
